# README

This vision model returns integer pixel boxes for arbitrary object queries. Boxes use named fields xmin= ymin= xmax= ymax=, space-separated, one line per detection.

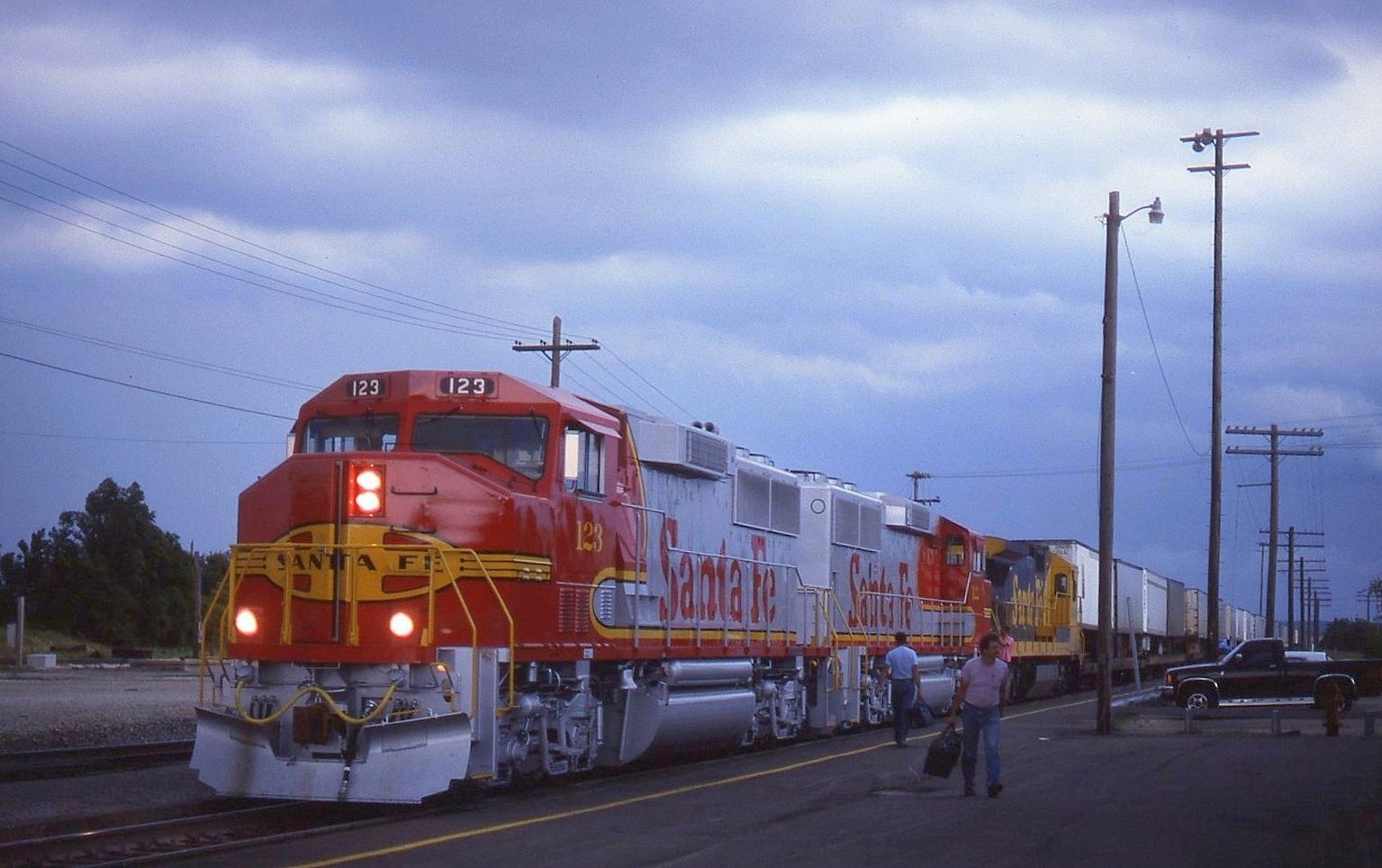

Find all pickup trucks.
xmin=1157 ymin=636 xmax=1382 ymax=709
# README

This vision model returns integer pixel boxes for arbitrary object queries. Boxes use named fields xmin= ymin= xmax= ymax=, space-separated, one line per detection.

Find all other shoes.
xmin=964 ymin=785 xmax=975 ymax=796
xmin=988 ymin=784 xmax=1003 ymax=797
xmin=895 ymin=736 xmax=907 ymax=748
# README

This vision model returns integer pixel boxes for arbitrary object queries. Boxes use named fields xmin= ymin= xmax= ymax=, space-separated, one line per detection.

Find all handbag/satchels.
xmin=913 ymin=697 xmax=934 ymax=728
xmin=923 ymin=724 xmax=961 ymax=778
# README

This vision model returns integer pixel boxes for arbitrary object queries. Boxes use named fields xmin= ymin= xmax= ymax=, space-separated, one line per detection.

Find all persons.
xmin=946 ymin=633 xmax=1009 ymax=798
xmin=995 ymin=627 xmax=1014 ymax=697
xmin=876 ymin=632 xmax=923 ymax=748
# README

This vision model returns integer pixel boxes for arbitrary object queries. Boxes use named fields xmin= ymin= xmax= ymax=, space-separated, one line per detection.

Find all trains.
xmin=188 ymin=367 xmax=1301 ymax=814
